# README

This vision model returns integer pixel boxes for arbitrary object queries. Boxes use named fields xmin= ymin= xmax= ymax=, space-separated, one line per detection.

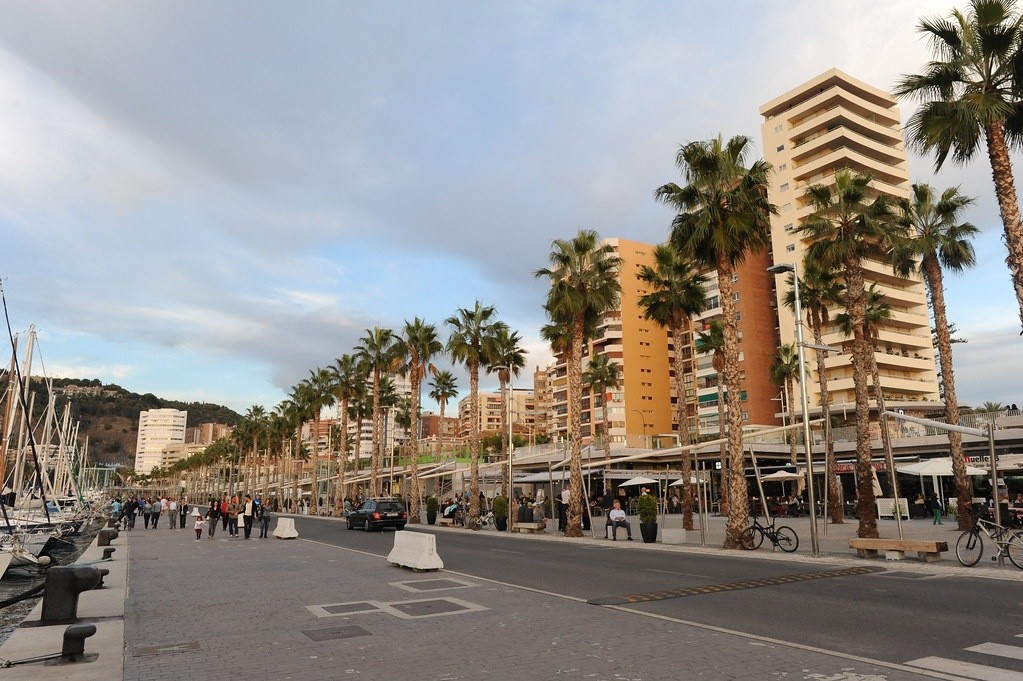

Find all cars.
xmin=345 ymin=496 xmax=408 ymax=532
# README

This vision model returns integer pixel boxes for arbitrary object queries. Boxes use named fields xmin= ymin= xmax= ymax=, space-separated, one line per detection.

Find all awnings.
xmin=507 ymin=468 xmax=604 ymax=484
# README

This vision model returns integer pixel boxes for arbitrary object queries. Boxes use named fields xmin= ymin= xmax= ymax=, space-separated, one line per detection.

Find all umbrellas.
xmin=617 ymin=476 xmax=660 ymax=496
xmin=760 ymin=470 xmax=806 ymax=496
xmin=896 ymin=458 xmax=988 ymax=510
xmin=668 ymin=476 xmax=708 ymax=486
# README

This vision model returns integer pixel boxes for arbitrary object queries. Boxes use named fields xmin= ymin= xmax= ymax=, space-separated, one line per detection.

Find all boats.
xmin=1 ymin=325 xmax=106 ymax=583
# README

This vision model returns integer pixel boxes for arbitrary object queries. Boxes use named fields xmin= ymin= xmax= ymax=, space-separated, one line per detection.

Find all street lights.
xmin=322 ymin=426 xmax=332 ymax=517
xmin=380 ymin=403 xmax=395 ymax=495
xmin=281 ymin=439 xmax=292 ymax=515
xmin=632 ymin=409 xmax=646 ymax=449
xmin=258 ymin=449 xmax=267 ymax=503
xmin=766 ymin=262 xmax=821 ymax=559
xmin=493 ymin=361 xmax=513 ymax=533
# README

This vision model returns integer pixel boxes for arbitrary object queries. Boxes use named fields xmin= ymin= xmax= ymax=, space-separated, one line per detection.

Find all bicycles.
xmin=469 ymin=504 xmax=498 ymax=532
xmin=124 ymin=519 xmax=131 ymax=530
xmin=956 ymin=507 xmax=1023 ymax=569
xmin=739 ymin=514 xmax=800 ymax=554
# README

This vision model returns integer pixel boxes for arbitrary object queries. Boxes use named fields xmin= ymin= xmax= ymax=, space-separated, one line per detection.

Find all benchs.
xmin=848 ymin=537 xmax=948 ymax=563
xmin=511 ymin=521 xmax=545 ymax=535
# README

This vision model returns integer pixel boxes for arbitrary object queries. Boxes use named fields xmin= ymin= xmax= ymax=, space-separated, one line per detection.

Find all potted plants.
xmin=945 ymin=503 xmax=958 ymax=523
xmin=636 ymin=495 xmax=659 ymax=544
xmin=892 ymin=502 xmax=906 ymax=520
xmin=426 ymin=498 xmax=438 ymax=525
xmin=494 ymin=497 xmax=508 ymax=531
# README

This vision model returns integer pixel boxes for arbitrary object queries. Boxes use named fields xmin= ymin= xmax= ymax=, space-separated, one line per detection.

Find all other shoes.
xmin=627 ymin=537 xmax=633 ymax=541
xmin=234 ymin=534 xmax=239 ymax=538
xmin=612 ymin=537 xmax=616 ymax=541
xmin=151 ymin=524 xmax=155 ymax=530
xmin=230 ymin=534 xmax=233 ymax=538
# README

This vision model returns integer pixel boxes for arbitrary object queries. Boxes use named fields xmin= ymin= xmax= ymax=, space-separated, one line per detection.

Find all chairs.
xmin=605 ymin=508 xmax=613 ymax=539
xmin=594 ymin=496 xmax=829 ymax=521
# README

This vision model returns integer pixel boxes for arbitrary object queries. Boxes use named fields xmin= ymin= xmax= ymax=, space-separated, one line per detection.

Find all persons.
xmin=694 ymin=495 xmax=698 ymax=501
xmin=914 ymin=494 xmax=924 ymax=504
xmin=671 ymin=494 xmax=679 ymax=514
xmin=751 ymin=496 xmax=800 ymax=516
xmin=111 ymin=497 xmax=188 ymax=531
xmin=446 ymin=488 xmax=634 ymax=541
xmin=205 ymin=494 xmax=274 ymax=540
xmin=343 ymin=493 xmax=411 ymax=517
xmin=714 ymin=492 xmax=722 ymax=511
xmin=657 ymin=496 xmax=669 ymax=508
xmin=930 ymin=493 xmax=944 ymax=525
xmin=989 ymin=494 xmax=1023 ymax=508
xmin=194 ymin=516 xmax=206 ymax=540
